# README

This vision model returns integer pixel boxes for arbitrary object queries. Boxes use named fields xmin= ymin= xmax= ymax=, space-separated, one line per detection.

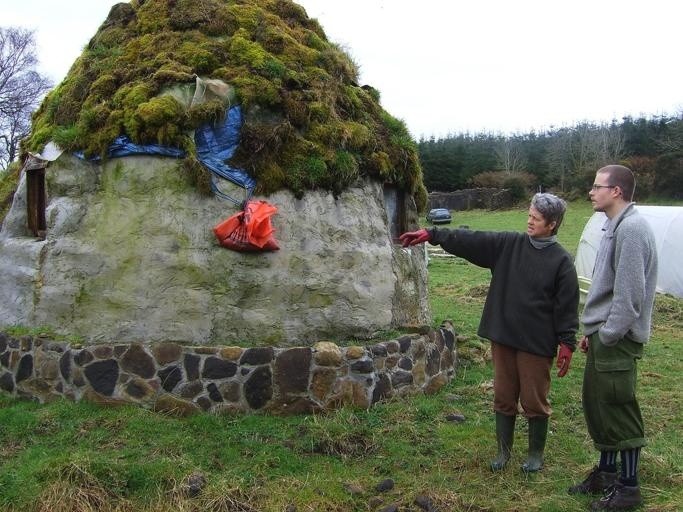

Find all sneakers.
xmin=590 ymin=484 xmax=642 ymax=511
xmin=567 ymin=469 xmax=617 ymax=494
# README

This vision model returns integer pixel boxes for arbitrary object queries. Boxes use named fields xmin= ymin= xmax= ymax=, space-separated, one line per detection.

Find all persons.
xmin=398 ymin=192 xmax=579 ymax=472
xmin=567 ymin=164 xmax=659 ymax=511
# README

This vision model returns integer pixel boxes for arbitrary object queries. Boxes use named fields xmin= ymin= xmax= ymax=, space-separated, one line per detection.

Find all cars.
xmin=426 ymin=208 xmax=452 ymax=225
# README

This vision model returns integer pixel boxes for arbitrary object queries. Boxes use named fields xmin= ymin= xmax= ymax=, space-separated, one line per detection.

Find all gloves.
xmin=556 ymin=343 xmax=572 ymax=377
xmin=400 ymin=228 xmax=429 ymax=247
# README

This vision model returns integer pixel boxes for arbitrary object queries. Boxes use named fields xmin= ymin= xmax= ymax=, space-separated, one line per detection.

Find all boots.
xmin=491 ymin=412 xmax=515 ymax=470
xmin=521 ymin=417 xmax=547 ymax=471
